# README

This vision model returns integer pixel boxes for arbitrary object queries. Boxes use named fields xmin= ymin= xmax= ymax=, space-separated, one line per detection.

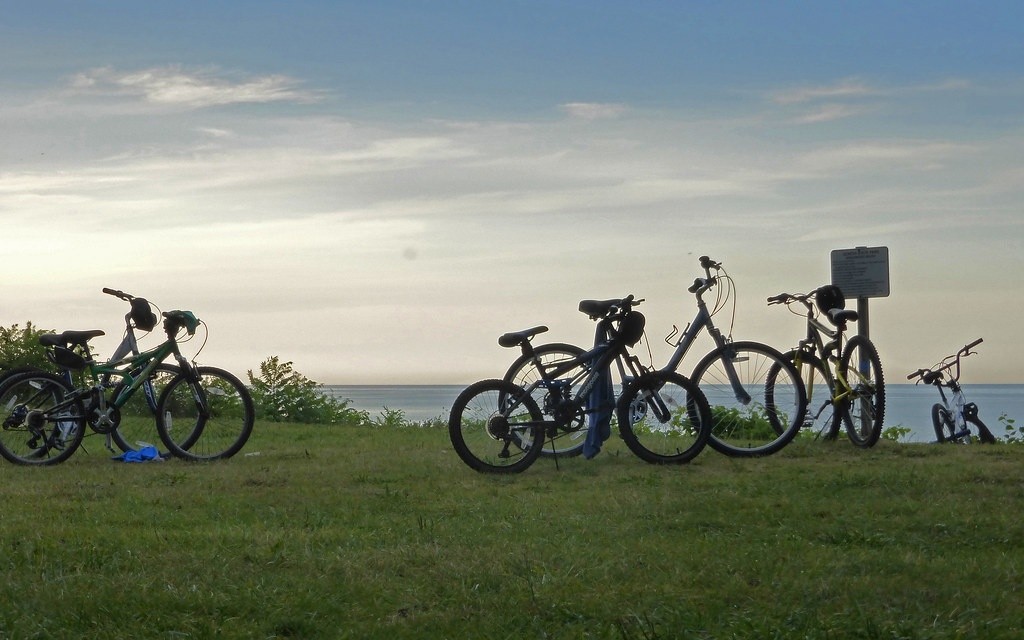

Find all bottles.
xmin=166 ymin=411 xmax=172 ymax=431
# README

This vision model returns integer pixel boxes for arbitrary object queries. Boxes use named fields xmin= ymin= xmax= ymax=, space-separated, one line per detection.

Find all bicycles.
xmin=0 ymin=287 xmax=255 ymax=466
xmin=764 ymin=284 xmax=885 ymax=449
xmin=907 ymin=338 xmax=995 ymax=444
xmin=497 ymin=256 xmax=807 ymax=458
xmin=449 ymin=295 xmax=712 ymax=475
